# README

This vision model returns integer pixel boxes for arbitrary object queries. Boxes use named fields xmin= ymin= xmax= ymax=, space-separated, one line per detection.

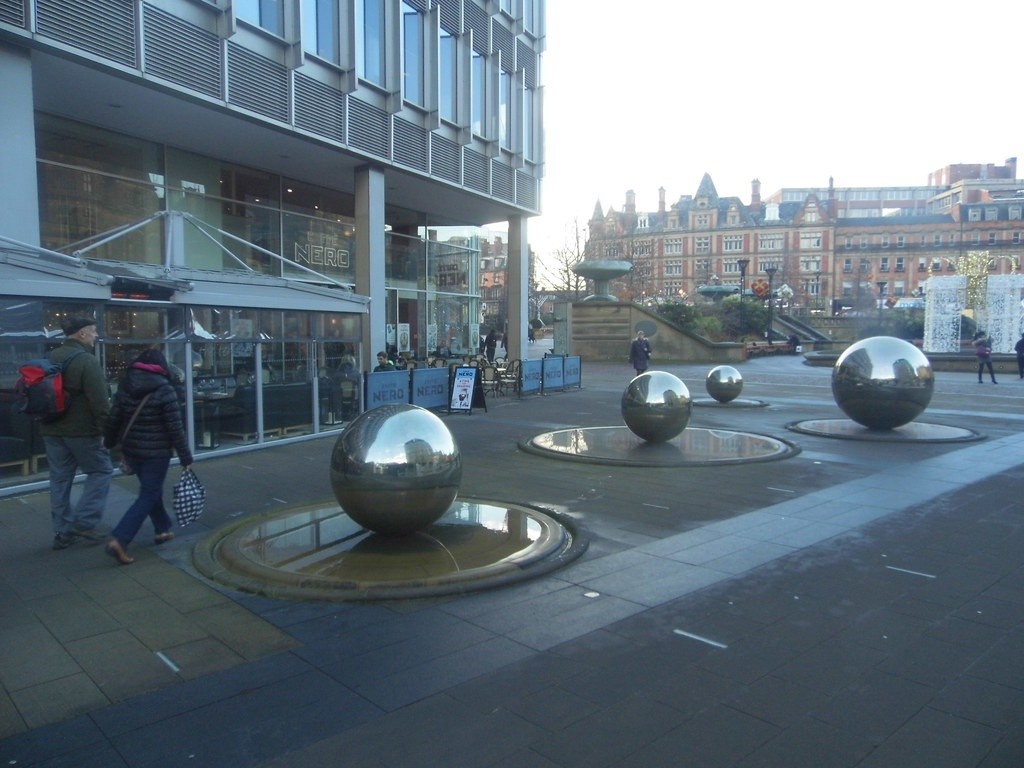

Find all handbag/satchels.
xmin=645 ymin=351 xmax=651 ymax=359
xmin=173 ymin=467 xmax=206 ymax=528
xmin=119 ymin=454 xmax=135 ymax=475
xmin=976 ymin=344 xmax=990 ymax=358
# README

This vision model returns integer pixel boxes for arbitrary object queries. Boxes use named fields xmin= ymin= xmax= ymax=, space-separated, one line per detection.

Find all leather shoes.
xmin=155 ymin=532 xmax=174 ymax=544
xmin=106 ymin=539 xmax=134 ymax=563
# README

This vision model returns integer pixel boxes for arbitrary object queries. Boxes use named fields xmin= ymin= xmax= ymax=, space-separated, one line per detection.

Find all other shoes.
xmin=72 ymin=527 xmax=105 ymax=544
xmin=52 ymin=532 xmax=80 ymax=548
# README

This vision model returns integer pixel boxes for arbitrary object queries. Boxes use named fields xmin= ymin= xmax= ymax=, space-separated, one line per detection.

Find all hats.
xmin=60 ymin=316 xmax=95 ymax=336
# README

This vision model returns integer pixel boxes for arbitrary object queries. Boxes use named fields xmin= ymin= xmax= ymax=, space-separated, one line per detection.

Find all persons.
xmin=374 ymin=336 xmax=462 ymax=371
xmin=104 ymin=349 xmax=194 ymax=564
xmin=484 ymin=330 xmax=497 ymax=362
xmin=628 ymin=330 xmax=650 ymax=376
xmin=1014 ymin=333 xmax=1024 ymax=378
xmin=322 ymin=355 xmax=363 ymax=425
xmin=41 ymin=317 xmax=114 ymax=548
xmin=528 ymin=323 xmax=536 ymax=342
xmin=787 ymin=334 xmax=800 ymax=353
xmin=970 ymin=331 xmax=998 ymax=384
xmin=500 ymin=332 xmax=509 ymax=360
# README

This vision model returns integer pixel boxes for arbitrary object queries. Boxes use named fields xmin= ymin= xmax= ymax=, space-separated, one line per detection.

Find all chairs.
xmin=233 ymin=353 xmax=522 ymax=422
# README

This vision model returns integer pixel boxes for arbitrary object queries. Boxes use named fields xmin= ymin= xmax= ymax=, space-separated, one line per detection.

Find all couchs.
xmin=30 ymin=414 xmax=49 ymax=474
xmin=192 ymin=373 xmax=312 ymax=445
xmin=0 ymin=393 xmax=30 ymax=476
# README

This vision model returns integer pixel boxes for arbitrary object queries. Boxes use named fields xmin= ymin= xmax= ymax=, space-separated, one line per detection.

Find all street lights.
xmin=737 ymin=260 xmax=750 ymax=338
xmin=764 ymin=268 xmax=780 ymax=343
xmin=877 ymin=281 xmax=887 ymax=323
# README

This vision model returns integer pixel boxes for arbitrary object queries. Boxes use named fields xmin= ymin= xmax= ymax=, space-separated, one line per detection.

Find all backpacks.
xmin=16 ymin=350 xmax=86 ymax=423
